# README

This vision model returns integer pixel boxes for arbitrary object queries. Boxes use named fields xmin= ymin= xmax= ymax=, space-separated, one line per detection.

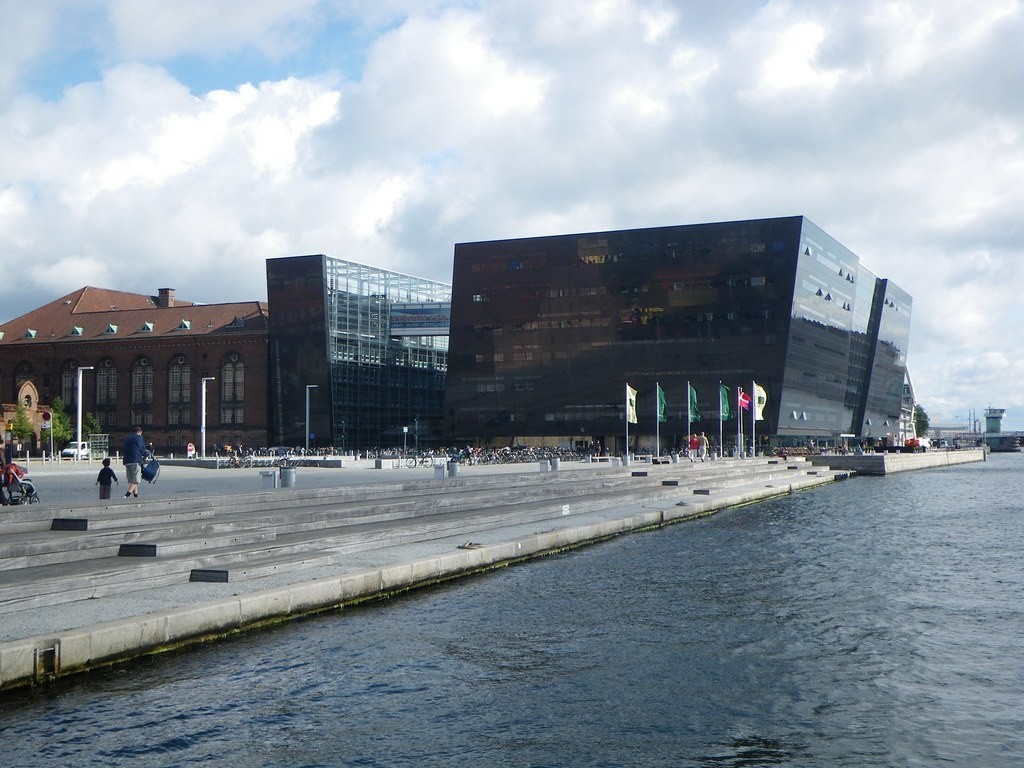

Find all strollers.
xmin=1 ymin=465 xmax=40 ymax=506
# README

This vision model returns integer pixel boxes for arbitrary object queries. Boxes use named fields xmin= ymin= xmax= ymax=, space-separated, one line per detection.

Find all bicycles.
xmin=450 ymin=447 xmax=581 ymax=465
xmin=272 ymin=458 xmax=287 ymax=467
xmin=405 ymin=452 xmax=434 ymax=468
xmin=642 ymin=445 xmax=789 ymax=458
xmin=228 ymin=455 xmax=251 ymax=468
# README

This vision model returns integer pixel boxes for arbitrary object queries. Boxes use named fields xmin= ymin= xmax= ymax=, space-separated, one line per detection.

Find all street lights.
xmin=78 ymin=366 xmax=94 ymax=460
xmin=201 ymin=377 xmax=215 ymax=458
xmin=306 ymin=384 xmax=318 ymax=451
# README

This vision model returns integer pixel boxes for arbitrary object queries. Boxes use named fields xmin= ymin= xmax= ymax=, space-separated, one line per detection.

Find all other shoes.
xmin=701 ymin=459 xmax=704 ymax=462
xmin=694 ymin=461 xmax=697 ymax=462
xmin=691 ymin=459 xmax=693 ymax=462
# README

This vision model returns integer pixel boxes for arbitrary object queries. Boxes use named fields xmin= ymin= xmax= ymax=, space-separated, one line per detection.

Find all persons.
xmin=368 ymin=445 xmax=475 ymax=466
xmin=589 ymin=440 xmax=601 ymax=457
xmin=0 ymin=434 xmax=9 ymax=506
xmin=96 ymin=458 xmax=119 ymax=499
xmin=212 ymin=444 xmax=305 ymax=457
xmin=689 ymin=432 xmax=708 ymax=463
xmin=770 ymin=439 xmax=865 ymax=460
xmin=123 ymin=426 xmax=155 ymax=498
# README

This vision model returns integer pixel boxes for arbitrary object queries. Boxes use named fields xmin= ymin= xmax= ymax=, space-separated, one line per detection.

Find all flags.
xmin=658 ymin=385 xmax=668 ymax=423
xmin=754 ymin=383 xmax=767 ymax=420
xmin=739 ymin=390 xmax=751 ymax=411
xmin=627 ymin=385 xmax=638 ymax=423
xmin=721 ymin=384 xmax=731 ymax=421
xmin=689 ymin=386 xmax=702 ymax=423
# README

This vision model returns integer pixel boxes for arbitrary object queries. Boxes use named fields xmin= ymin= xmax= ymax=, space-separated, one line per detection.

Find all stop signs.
xmin=188 ymin=444 xmax=193 ymax=451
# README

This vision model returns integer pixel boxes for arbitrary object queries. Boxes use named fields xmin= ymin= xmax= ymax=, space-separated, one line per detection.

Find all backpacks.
xmin=141 ymin=452 xmax=160 ymax=484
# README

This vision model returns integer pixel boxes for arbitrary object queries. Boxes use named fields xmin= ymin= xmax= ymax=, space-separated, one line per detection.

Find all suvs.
xmin=62 ymin=442 xmax=91 ymax=457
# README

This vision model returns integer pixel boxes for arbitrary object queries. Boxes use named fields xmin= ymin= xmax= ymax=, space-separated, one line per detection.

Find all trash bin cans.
xmin=550 ymin=458 xmax=560 ymax=470
xmin=741 ymin=452 xmax=746 ymax=460
xmin=449 ymin=461 xmax=460 ymax=476
xmin=280 ymin=467 xmax=296 ymax=487
